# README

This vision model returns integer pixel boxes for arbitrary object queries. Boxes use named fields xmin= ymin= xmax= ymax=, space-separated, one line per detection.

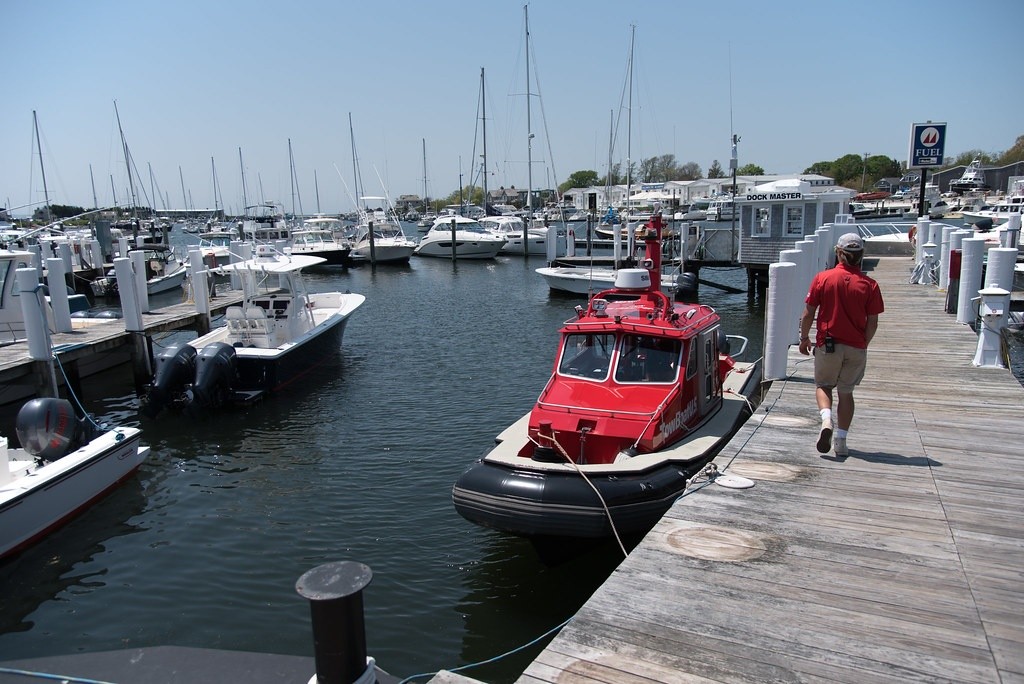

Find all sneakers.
xmin=817 ymin=423 xmax=833 ymax=453
xmin=833 ymin=435 xmax=849 ymax=456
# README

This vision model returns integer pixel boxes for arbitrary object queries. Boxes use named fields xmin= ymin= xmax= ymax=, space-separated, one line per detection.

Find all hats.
xmin=834 ymin=233 xmax=863 ymax=251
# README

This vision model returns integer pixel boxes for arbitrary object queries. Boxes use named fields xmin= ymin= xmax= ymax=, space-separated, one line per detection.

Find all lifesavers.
xmin=909 ymin=225 xmax=917 ymax=244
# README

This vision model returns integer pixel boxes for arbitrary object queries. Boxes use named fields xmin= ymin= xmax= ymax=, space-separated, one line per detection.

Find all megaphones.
xmin=640 ymin=258 xmax=653 ymax=268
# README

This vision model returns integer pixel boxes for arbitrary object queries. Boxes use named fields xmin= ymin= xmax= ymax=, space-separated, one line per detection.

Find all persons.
xmin=973 ymin=217 xmax=998 ymax=233
xmin=799 ymin=233 xmax=884 ymax=455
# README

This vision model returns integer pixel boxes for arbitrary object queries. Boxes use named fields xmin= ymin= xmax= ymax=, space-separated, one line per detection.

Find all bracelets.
xmin=800 ymin=336 xmax=809 ymax=342
